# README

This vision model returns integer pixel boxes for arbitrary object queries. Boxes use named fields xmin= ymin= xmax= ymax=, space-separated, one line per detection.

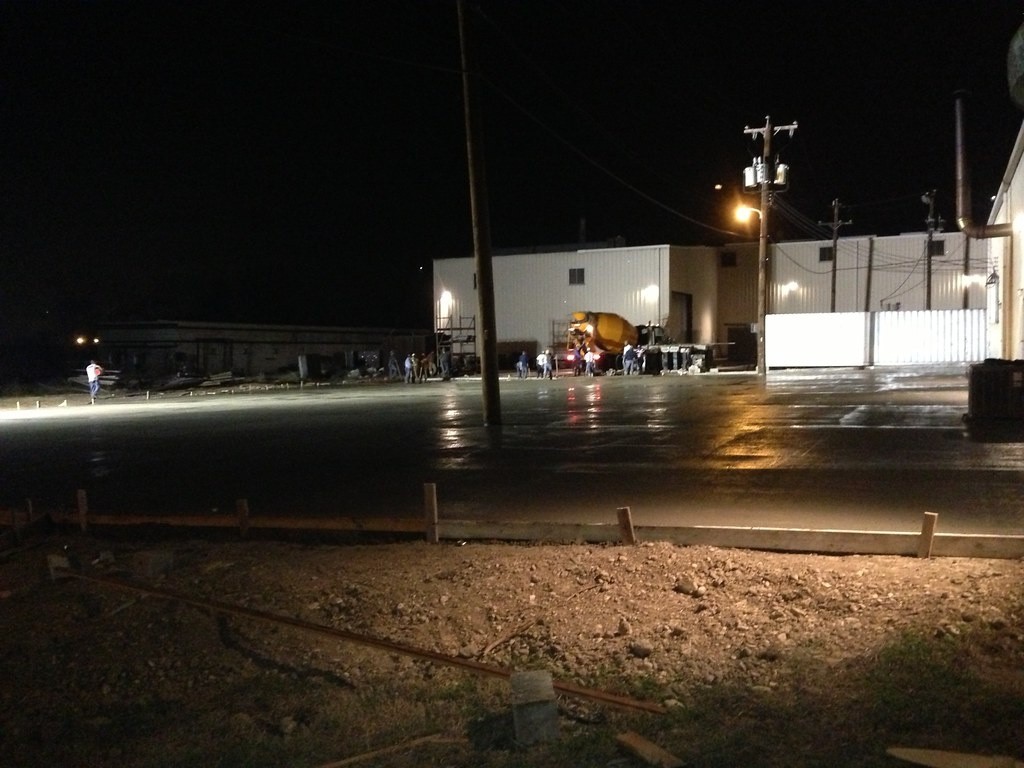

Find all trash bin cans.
xmin=967 ymin=363 xmax=1024 ymax=441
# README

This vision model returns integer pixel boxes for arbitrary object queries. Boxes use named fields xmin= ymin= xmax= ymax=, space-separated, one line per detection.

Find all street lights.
xmin=734 ymin=204 xmax=767 ymax=373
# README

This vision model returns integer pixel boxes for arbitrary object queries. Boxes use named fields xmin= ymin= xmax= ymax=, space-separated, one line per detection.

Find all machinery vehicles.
xmin=568 ymin=312 xmax=672 ymax=370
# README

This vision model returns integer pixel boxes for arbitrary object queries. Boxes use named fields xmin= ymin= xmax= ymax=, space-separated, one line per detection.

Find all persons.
xmin=622 ymin=340 xmax=645 ymax=375
xmin=514 ymin=349 xmax=554 ymax=380
xmin=584 ymin=348 xmax=594 ymax=376
xmin=86 ymin=360 xmax=102 ymax=404
xmin=574 ymin=341 xmax=584 ymax=376
xmin=389 ymin=348 xmax=450 ymax=384
